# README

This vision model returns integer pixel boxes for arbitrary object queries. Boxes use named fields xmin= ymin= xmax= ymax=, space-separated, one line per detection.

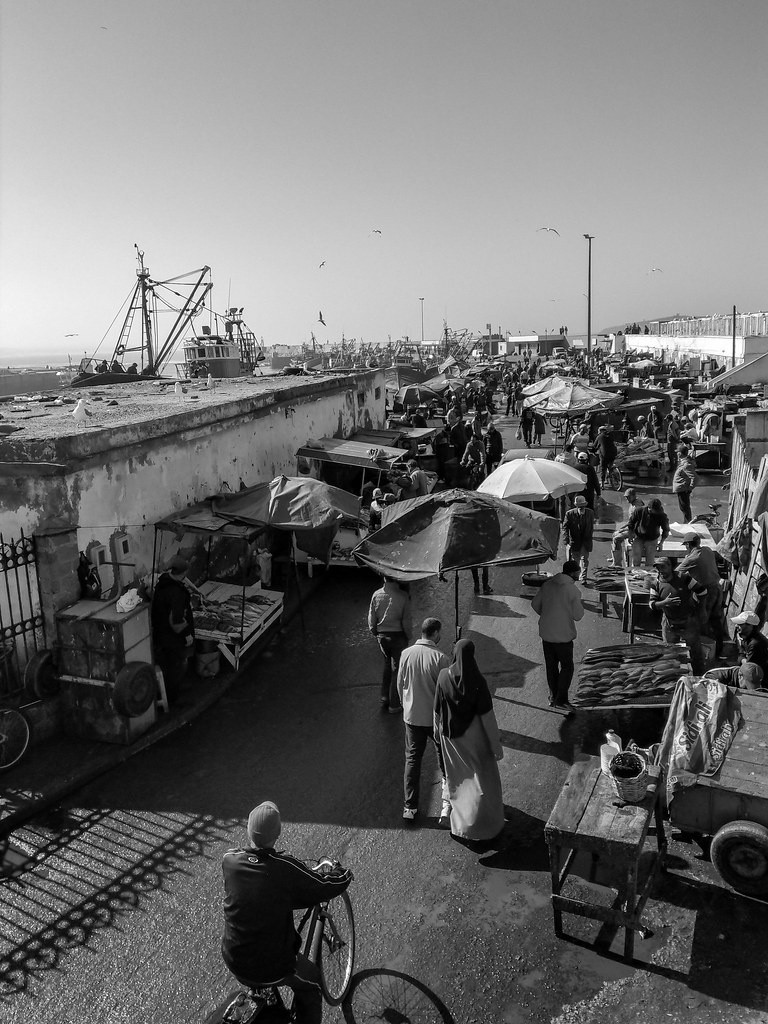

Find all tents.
xmin=147 ymin=493 xmax=268 ymax=644
xmin=295 ymin=433 xmax=412 ymax=488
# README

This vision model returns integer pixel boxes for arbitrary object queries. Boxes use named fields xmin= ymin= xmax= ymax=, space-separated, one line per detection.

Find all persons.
xmin=394 ymin=618 xmax=447 ymax=822
xmin=559 ymin=494 xmax=596 ymax=584
xmin=431 ymin=637 xmax=505 ymax=846
xmin=152 ymin=556 xmax=201 ymax=684
xmin=218 ymin=799 xmax=354 ymax=1024
xmin=398 ymin=322 xmax=768 ymax=485
xmin=553 ymin=421 xmax=618 ymax=512
xmin=359 ymin=457 xmax=429 ymax=526
xmin=671 ymin=444 xmax=698 ymax=522
xmin=531 ymin=560 xmax=585 ymax=711
xmin=366 ymin=576 xmax=410 ymax=713
xmin=611 ymin=489 xmax=768 ymax=690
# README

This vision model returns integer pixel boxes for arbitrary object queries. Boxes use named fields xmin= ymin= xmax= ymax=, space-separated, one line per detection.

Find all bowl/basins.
xmin=417 ymin=443 xmax=426 ymax=454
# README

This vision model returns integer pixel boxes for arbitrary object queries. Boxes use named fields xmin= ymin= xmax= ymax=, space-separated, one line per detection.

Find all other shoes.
xmin=582 ymin=579 xmax=587 ymax=585
xmin=379 ymin=696 xmax=388 ymax=703
xmin=547 ymin=695 xmax=573 ymax=711
xmin=388 ymin=707 xmax=402 ymax=713
xmin=403 ymin=807 xmax=417 ymax=819
xmin=438 ymin=808 xmax=450 ymax=829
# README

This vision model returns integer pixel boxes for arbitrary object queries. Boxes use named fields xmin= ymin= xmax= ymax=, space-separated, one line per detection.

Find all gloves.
xmin=184 ymin=633 xmax=193 ymax=646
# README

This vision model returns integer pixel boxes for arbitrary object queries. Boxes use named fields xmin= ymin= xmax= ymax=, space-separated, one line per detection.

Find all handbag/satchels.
xmin=669 ymin=623 xmax=688 ymax=635
xmin=77 ymin=550 xmax=101 ymax=599
xmin=516 ymin=427 xmax=522 ymax=440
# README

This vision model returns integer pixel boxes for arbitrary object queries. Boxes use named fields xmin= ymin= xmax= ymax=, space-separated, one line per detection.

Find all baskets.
xmin=610 ymin=751 xmax=646 ymax=801
xmin=588 ymin=454 xmax=599 ymax=466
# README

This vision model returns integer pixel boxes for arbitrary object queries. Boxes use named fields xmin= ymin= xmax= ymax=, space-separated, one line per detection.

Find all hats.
xmin=563 ymin=561 xmax=580 ymax=573
xmin=574 ymin=496 xmax=587 ymax=506
xmin=372 ymin=488 xmax=382 ymax=499
xmin=249 ymin=801 xmax=281 ymax=844
xmin=682 ymin=532 xmax=700 ymax=545
xmin=730 ymin=611 xmax=760 ymax=625
xmin=578 ymin=452 xmax=588 ymax=459
xmin=624 ymin=488 xmax=636 ymax=496
xmin=674 ymin=446 xmax=688 ymax=452
xmin=740 ymin=662 xmax=763 ymax=689
xmin=653 ymin=557 xmax=670 ymax=567
xmin=171 ymin=559 xmax=190 ymax=573
xmin=379 ymin=493 xmax=396 ymax=501
xmin=664 ymin=414 xmax=673 ymax=420
xmin=649 ymin=498 xmax=663 ymax=514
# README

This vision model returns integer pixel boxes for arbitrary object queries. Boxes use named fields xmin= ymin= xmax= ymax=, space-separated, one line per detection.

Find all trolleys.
xmin=666 ymin=681 xmax=768 ymax=899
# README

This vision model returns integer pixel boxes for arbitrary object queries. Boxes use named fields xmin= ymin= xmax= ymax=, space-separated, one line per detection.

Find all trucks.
xmin=552 ymin=347 xmax=567 ymax=359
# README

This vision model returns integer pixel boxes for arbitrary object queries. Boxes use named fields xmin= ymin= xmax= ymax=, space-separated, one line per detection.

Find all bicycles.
xmin=687 ymin=503 xmax=722 ymax=529
xmin=463 ymin=464 xmax=484 ymax=491
xmin=0 ymin=704 xmax=33 ymax=774
xmin=549 ymin=416 xmax=566 ymax=428
xmin=223 ymin=857 xmax=356 ymax=1024
xmin=588 ymin=445 xmax=623 ymax=492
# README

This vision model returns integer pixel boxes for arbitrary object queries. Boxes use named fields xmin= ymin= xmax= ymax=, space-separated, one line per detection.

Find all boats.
xmin=267 ymin=318 xmax=473 ymax=376
xmin=56 ymin=243 xmax=266 ymax=387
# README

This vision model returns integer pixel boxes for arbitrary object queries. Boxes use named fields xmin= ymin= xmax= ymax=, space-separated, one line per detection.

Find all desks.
xmin=692 ymin=442 xmax=726 ymax=466
xmin=307 ymin=527 xmax=370 ymax=577
xmin=545 ymin=752 xmax=666 ymax=962
xmin=427 ymin=477 xmax=438 ymax=493
xmin=574 ymin=521 xmax=718 ymax=734
xmin=191 ymin=579 xmax=284 ymax=671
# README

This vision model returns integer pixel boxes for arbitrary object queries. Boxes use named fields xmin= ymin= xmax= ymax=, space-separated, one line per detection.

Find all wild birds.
xmin=536 ymin=227 xmax=559 ymax=236
xmin=72 ymin=399 xmax=90 ymax=428
xmin=174 ymin=382 xmax=182 ymax=402
xmin=318 ymin=311 xmax=326 ymax=326
xmin=207 ymin=373 xmax=215 ymax=394
xmin=649 ymin=268 xmax=663 ymax=272
xmin=65 ymin=334 xmax=78 ymax=337
xmin=373 ymin=230 xmax=381 ymax=233
xmin=320 ymin=261 xmax=326 ymax=268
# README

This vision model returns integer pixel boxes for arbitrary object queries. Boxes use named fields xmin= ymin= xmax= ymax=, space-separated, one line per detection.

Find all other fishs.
xmin=208 ymin=595 xmax=276 ymax=633
xmin=574 ymin=642 xmax=691 ymax=698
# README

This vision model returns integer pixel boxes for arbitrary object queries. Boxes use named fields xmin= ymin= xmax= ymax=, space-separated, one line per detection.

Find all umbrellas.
xmin=397 ymin=377 xmax=442 ymax=406
xmin=349 ymin=487 xmax=562 ymax=647
xmin=212 ymin=475 xmax=362 ymax=636
xmin=478 ymin=455 xmax=590 ymax=513
xmin=520 ymin=380 xmax=626 ymax=413
xmin=522 ymin=368 xmax=593 ymax=398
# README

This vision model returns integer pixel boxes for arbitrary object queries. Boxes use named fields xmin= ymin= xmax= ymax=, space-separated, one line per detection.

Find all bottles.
xmin=606 ymin=729 xmax=622 ymax=753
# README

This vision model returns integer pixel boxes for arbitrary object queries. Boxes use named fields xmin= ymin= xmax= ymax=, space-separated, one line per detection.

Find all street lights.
xmin=419 ymin=297 xmax=424 ymax=342
xmin=583 ymin=233 xmax=595 ymax=356
